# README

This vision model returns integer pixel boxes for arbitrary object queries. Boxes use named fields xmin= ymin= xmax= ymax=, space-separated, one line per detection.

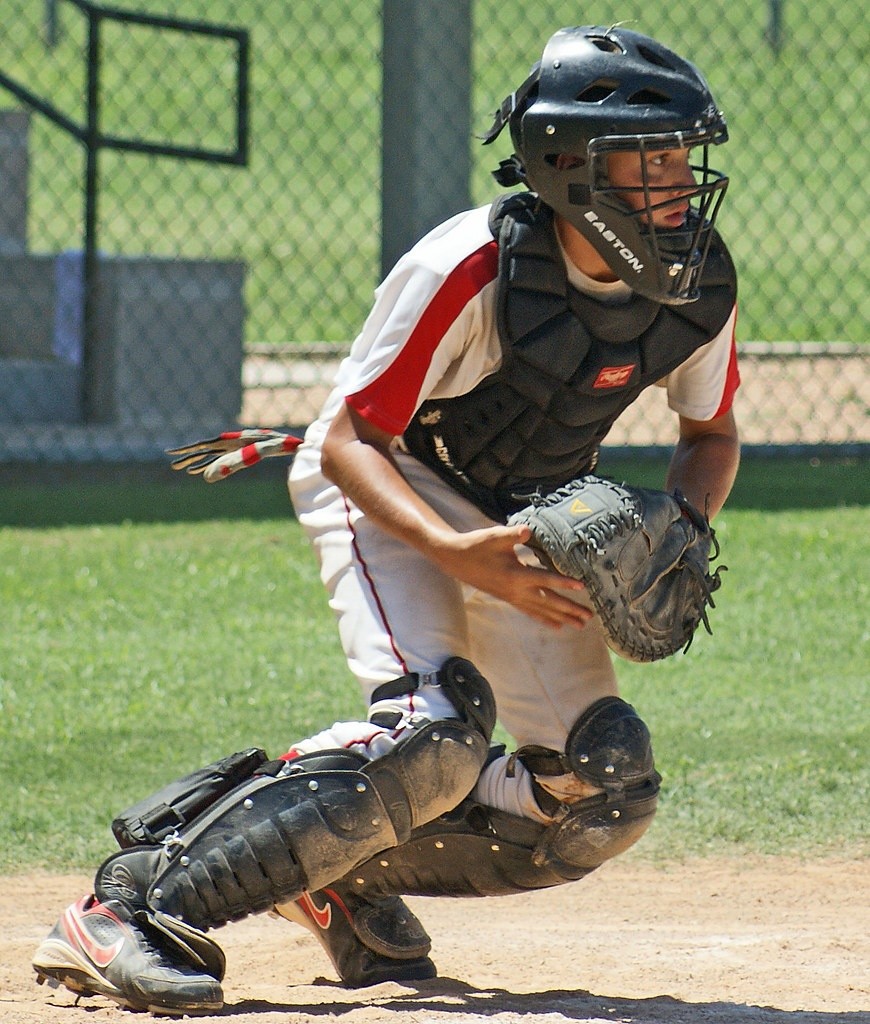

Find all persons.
xmin=33 ymin=25 xmax=743 ymax=1014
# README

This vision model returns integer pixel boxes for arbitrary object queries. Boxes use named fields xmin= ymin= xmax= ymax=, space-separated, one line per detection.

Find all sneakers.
xmin=31 ymin=892 xmax=225 ymax=1016
xmin=267 ymin=888 xmax=438 ymax=989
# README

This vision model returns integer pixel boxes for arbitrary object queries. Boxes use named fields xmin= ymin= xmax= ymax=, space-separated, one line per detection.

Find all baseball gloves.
xmin=506 ymin=472 xmax=732 ymax=664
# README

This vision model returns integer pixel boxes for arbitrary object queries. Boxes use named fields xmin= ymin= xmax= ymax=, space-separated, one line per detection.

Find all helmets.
xmin=508 ymin=26 xmax=730 ymax=306
